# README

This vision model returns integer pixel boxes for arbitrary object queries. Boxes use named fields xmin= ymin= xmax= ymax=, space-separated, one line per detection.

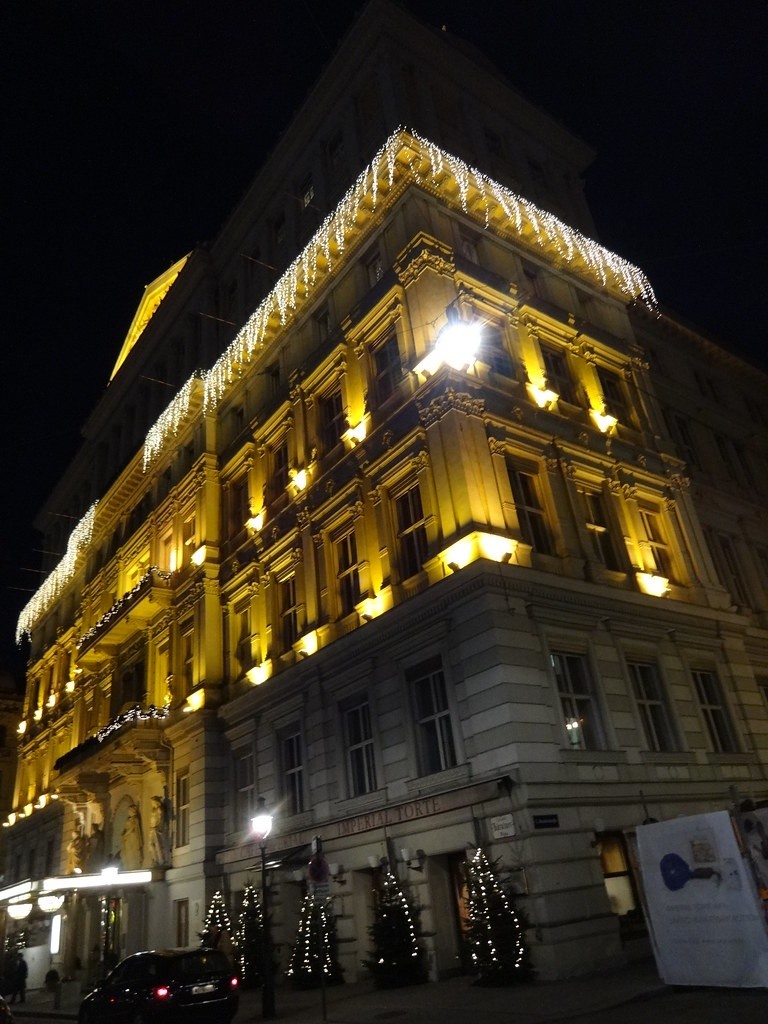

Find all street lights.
xmin=249 ymin=796 xmax=277 ymax=1024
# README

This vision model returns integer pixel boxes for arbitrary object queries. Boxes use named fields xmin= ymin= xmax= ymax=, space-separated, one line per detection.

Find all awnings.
xmin=245 ymin=843 xmax=310 ymax=876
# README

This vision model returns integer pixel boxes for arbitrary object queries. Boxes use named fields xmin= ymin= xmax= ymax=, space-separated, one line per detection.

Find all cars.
xmin=76 ymin=945 xmax=241 ymax=1024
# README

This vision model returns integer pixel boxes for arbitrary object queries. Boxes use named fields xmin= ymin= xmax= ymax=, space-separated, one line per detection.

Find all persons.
xmin=147 ymin=795 xmax=168 ymax=868
xmin=85 ymin=823 xmax=105 ymax=873
xmin=118 ymin=805 xmax=144 ymax=870
xmin=66 ymin=831 xmax=88 ymax=876
xmin=7 ymin=953 xmax=28 ymax=1004
